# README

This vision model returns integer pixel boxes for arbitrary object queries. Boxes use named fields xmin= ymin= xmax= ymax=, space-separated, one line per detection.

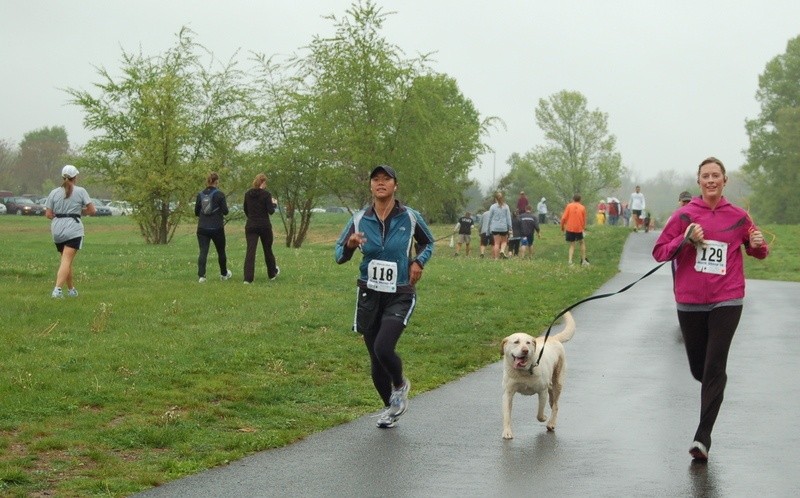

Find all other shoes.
xmin=480 ymin=252 xmax=485 ymax=258
xmin=220 ymin=269 xmax=232 ymax=281
xmin=68 ymin=288 xmax=78 ymax=297
xmin=581 ymin=258 xmax=591 ymax=265
xmin=455 ymin=252 xmax=459 ymax=256
xmin=270 ymin=266 xmax=280 ymax=280
xmin=52 ymin=288 xmax=63 ymax=298
xmin=689 ymin=441 xmax=708 ymax=460
xmin=243 ymin=280 xmax=250 ymax=284
xmin=500 ymin=251 xmax=507 ymax=258
xmin=199 ymin=277 xmax=206 ymax=283
xmin=509 ymin=255 xmax=513 ymax=260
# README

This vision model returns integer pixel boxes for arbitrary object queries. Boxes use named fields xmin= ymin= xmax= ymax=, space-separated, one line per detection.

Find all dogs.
xmin=501 ymin=311 xmax=577 ymax=439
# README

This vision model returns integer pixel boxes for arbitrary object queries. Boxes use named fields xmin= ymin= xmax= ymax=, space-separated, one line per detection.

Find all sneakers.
xmin=388 ymin=376 xmax=411 ymax=417
xmin=376 ymin=406 xmax=400 ymax=428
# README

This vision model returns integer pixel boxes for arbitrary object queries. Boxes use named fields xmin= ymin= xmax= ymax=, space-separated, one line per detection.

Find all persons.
xmin=243 ymin=174 xmax=280 ymax=285
xmin=596 ymin=185 xmax=655 ymax=234
xmin=476 ymin=190 xmax=561 ymax=260
xmin=46 ymin=165 xmax=97 ymax=299
xmin=335 ymin=164 xmax=434 ymax=430
xmin=194 ymin=173 xmax=232 ymax=283
xmin=449 ymin=212 xmax=474 ymax=259
xmin=487 ymin=190 xmax=512 ymax=261
xmin=652 ymin=157 xmax=772 ymax=459
xmin=560 ymin=194 xmax=592 ymax=270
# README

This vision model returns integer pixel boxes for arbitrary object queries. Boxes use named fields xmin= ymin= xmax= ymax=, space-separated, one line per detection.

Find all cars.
xmin=327 ymin=207 xmax=355 ymax=215
xmin=274 ymin=205 xmax=326 ymax=214
xmin=0 ymin=190 xmax=48 ymax=216
xmin=155 ymin=201 xmax=199 ymax=215
xmin=81 ymin=197 xmax=140 ymax=216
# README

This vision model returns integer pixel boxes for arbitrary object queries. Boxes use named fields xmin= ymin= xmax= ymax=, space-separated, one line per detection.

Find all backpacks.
xmin=199 ymin=189 xmax=221 ymax=216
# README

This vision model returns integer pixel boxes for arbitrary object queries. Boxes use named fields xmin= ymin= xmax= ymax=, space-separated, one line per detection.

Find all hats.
xmin=62 ymin=165 xmax=79 ymax=178
xmin=679 ymin=191 xmax=693 ymax=201
xmin=370 ymin=165 xmax=396 ymax=178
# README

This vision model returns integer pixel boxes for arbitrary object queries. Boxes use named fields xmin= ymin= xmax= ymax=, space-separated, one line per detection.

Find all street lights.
xmin=493 ymin=150 xmax=496 ymax=190
